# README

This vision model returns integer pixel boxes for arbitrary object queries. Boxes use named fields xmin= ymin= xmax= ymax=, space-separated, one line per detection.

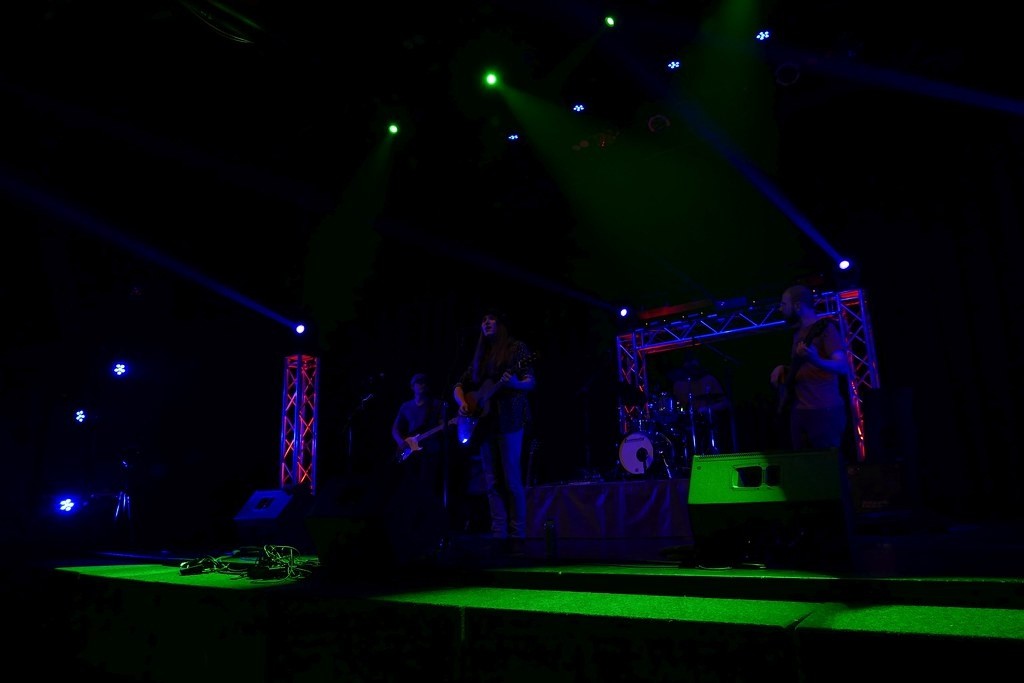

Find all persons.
xmin=771 ymin=285 xmax=879 ymax=572
xmin=452 ymin=312 xmax=535 ymax=539
xmin=392 ymin=372 xmax=447 ymax=469
xmin=347 ymin=374 xmax=393 ymax=490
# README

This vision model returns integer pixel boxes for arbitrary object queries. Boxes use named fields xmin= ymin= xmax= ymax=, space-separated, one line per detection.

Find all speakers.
xmin=233 ymin=490 xmax=301 ymax=548
xmin=687 ymin=446 xmax=839 ymax=565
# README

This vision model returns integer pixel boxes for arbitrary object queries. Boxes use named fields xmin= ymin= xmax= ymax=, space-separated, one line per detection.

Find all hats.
xmin=410 ymin=373 xmax=427 ymax=386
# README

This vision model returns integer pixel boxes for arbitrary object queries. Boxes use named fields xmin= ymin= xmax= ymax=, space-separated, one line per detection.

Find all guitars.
xmin=457 ymin=351 xmax=536 ymax=449
xmin=776 ymin=320 xmax=827 ymax=415
xmin=395 ymin=416 xmax=458 ymax=463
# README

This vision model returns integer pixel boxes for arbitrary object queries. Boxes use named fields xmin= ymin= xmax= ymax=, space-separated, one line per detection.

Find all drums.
xmin=619 ymin=430 xmax=673 ymax=475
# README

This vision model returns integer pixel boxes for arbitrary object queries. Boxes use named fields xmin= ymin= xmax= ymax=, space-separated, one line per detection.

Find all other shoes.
xmin=504 ymin=536 xmax=526 ymax=550
xmin=484 ymin=537 xmax=504 ymax=551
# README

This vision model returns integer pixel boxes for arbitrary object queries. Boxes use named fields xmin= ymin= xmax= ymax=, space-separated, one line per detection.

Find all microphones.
xmin=442 ymin=401 xmax=449 ymax=420
